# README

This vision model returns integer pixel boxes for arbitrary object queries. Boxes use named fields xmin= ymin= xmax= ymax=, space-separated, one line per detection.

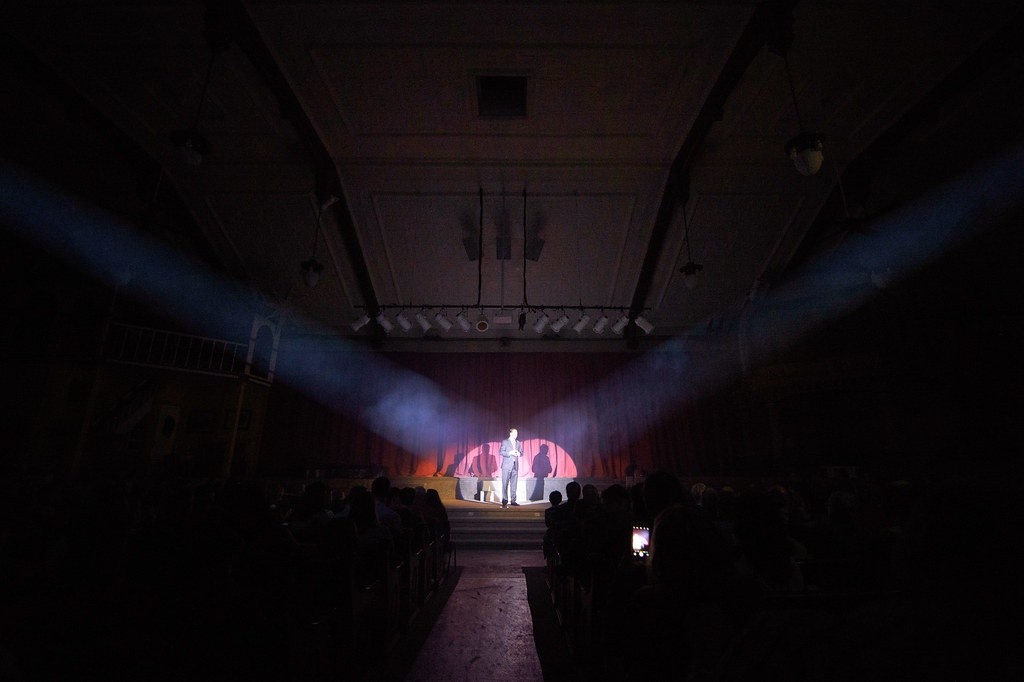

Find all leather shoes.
xmin=501 ymin=499 xmax=519 ymax=507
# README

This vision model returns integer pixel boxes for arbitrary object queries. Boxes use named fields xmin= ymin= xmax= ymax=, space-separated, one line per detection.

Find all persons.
xmin=3 ymin=465 xmax=452 ymax=682
xmin=545 ymin=460 xmax=1024 ymax=682
xmin=499 ymin=428 xmax=522 ymax=507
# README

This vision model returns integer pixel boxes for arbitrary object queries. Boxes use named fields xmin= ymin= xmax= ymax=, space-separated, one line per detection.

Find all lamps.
xmin=549 ymin=316 xmax=570 ymax=333
xmin=350 ymin=314 xmax=371 ymax=333
xmin=593 ymin=315 xmax=610 ymax=333
xmin=376 ymin=313 xmax=394 ymax=333
xmin=414 ymin=312 xmax=433 ymax=332
xmin=635 ymin=315 xmax=656 ymax=335
xmin=571 ymin=316 xmax=591 ymax=332
xmin=394 ymin=315 xmax=413 ymax=332
xmin=475 ymin=316 xmax=490 ymax=332
xmin=783 ymin=129 xmax=831 ymax=177
xmin=299 ymin=260 xmax=319 ymax=288
xmin=533 ymin=315 xmax=549 ymax=335
xmin=169 ymin=125 xmax=214 ymax=168
xmin=611 ymin=315 xmax=630 ymax=335
xmin=679 ymin=260 xmax=706 ymax=289
xmin=456 ymin=314 xmax=472 ymax=331
xmin=434 ymin=312 xmax=454 ymax=331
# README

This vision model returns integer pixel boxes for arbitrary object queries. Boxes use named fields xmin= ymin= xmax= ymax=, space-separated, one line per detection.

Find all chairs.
xmin=540 ymin=532 xmax=629 ymax=659
xmin=280 ymin=531 xmax=459 ymax=668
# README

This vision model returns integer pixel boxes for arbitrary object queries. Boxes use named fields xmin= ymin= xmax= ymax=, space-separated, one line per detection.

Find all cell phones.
xmin=631 ymin=524 xmax=651 ymax=560
